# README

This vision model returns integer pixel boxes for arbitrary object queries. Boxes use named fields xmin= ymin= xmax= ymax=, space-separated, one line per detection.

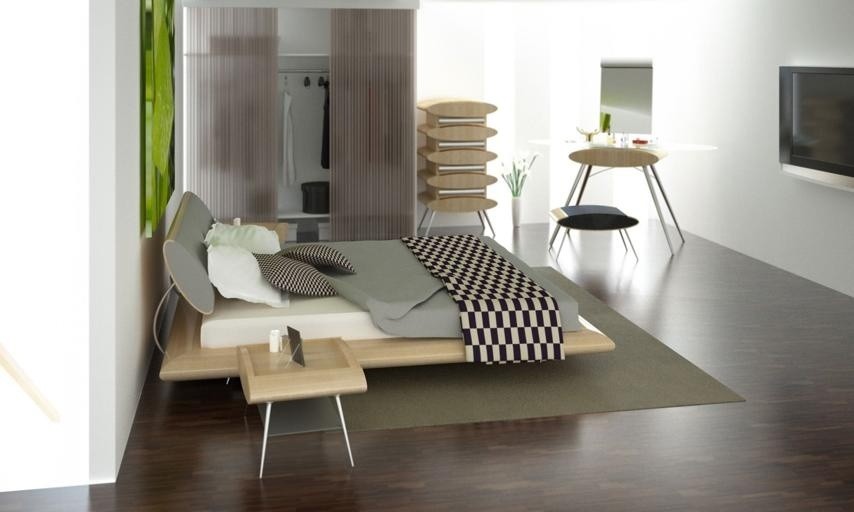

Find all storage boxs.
xmin=316 ymin=222 xmax=331 ymax=242
xmin=286 ymin=223 xmax=298 ymax=242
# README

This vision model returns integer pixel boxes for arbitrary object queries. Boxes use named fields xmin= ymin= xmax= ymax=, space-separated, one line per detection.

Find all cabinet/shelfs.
xmin=176 ymin=0 xmax=416 ymax=241
xmin=415 ymin=97 xmax=499 ymax=236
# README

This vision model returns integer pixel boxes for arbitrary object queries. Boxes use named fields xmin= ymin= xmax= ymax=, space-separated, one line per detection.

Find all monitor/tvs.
xmin=778 ymin=66 xmax=854 ymax=193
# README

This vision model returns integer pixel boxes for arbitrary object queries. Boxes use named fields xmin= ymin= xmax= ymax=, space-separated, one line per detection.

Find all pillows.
xmin=252 ymin=251 xmax=337 ymax=298
xmin=274 ymin=243 xmax=357 ymax=275
xmin=203 ymin=223 xmax=290 ymax=309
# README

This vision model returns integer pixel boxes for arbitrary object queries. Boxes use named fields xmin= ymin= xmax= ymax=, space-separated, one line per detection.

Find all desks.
xmin=549 ymin=146 xmax=685 ymax=256
xmin=235 ymin=336 xmax=368 ymax=480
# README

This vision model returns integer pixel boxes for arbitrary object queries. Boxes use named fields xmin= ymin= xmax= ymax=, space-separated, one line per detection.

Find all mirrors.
xmin=598 ymin=66 xmax=653 ymax=133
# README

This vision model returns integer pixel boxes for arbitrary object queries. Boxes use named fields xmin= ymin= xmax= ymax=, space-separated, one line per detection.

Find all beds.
xmin=151 ymin=190 xmax=616 ymax=387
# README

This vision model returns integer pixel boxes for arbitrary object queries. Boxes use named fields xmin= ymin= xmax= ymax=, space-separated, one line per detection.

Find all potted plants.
xmin=500 ymin=153 xmax=537 ymax=228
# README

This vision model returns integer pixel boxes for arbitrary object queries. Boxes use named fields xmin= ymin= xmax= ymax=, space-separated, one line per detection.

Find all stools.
xmin=549 ymin=205 xmax=641 ymax=262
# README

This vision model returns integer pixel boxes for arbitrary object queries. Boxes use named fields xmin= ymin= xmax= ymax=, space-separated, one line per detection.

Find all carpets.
xmin=257 ymin=265 xmax=747 ymax=437
